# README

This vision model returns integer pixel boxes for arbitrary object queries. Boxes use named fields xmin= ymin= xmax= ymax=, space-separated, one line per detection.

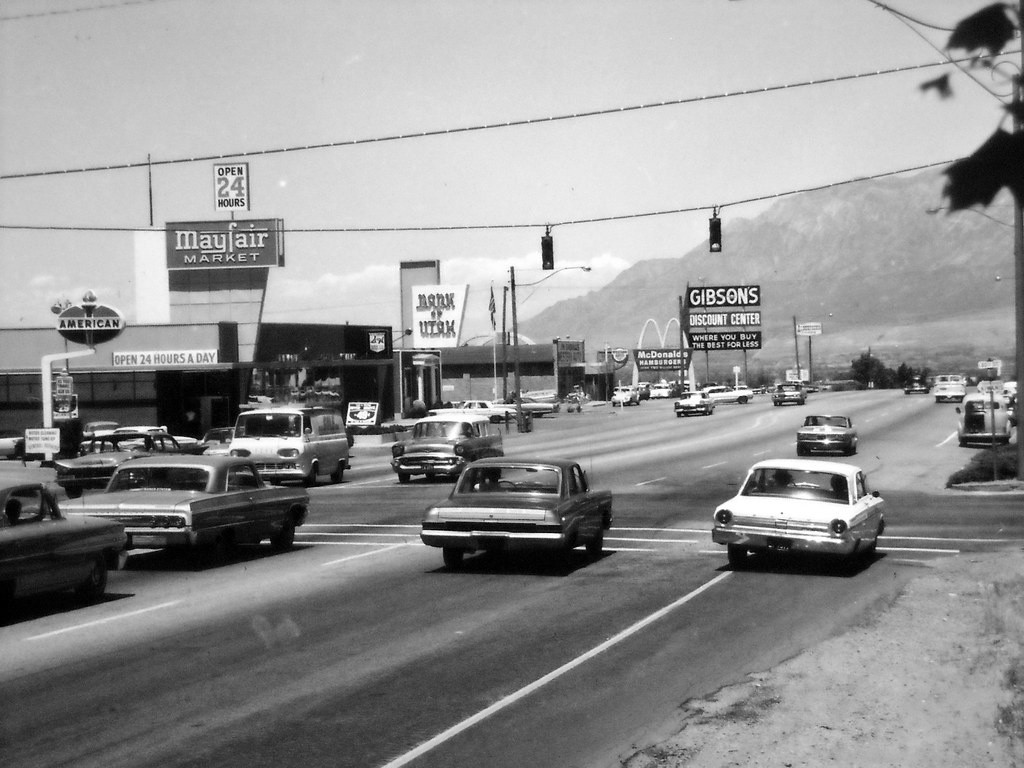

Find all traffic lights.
xmin=709 ymin=218 xmax=722 ymax=253
xmin=541 ymin=236 xmax=554 ymax=270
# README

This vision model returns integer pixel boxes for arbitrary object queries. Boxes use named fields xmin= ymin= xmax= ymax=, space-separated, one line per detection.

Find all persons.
xmin=831 ymin=475 xmax=848 ymax=499
xmin=774 ymin=471 xmax=792 ymax=488
xmin=460 ymin=424 xmax=471 ymax=437
xmin=475 ymin=469 xmax=501 ymax=491
xmin=811 ymin=417 xmax=818 ymax=425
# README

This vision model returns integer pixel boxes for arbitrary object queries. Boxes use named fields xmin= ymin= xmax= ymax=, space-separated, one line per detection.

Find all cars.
xmin=902 ymin=374 xmax=1017 ymax=447
xmin=610 ymin=378 xmax=809 ymax=407
xmin=796 ymin=415 xmax=858 ymax=456
xmin=444 ymin=387 xmax=561 ymax=424
xmin=0 ymin=419 xmax=245 ymax=499
xmin=388 ymin=412 xmax=505 ymax=484
xmin=417 ymin=456 xmax=613 ymax=572
xmin=711 ymin=457 xmax=887 ymax=573
xmin=55 ymin=453 xmax=311 ymax=566
xmin=673 ymin=391 xmax=716 ymax=417
xmin=0 ymin=474 xmax=128 ymax=606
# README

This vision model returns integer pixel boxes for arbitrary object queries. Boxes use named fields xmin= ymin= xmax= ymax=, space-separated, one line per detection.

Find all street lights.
xmin=927 ymin=206 xmax=1024 ymax=483
xmin=508 ymin=265 xmax=592 ymax=433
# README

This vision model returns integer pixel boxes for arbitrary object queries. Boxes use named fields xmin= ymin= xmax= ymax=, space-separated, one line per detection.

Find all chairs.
xmin=272 ymin=417 xmax=289 ymax=433
xmin=830 ymin=474 xmax=847 ymax=501
xmin=767 ymin=469 xmax=793 ymax=489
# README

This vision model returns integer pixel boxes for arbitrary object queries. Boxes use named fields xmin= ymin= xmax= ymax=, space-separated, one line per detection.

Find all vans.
xmin=227 ymin=404 xmax=352 ymax=487
